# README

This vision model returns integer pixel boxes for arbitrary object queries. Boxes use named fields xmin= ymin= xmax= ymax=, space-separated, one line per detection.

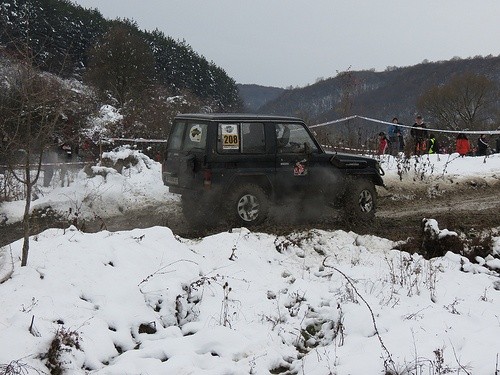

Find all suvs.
xmin=163 ymin=114 xmax=386 ymax=226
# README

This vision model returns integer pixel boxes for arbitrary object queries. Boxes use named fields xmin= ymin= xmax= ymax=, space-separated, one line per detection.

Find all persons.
xmin=274 ymin=123 xmax=291 ymax=149
xmin=42 ymin=137 xmax=72 ymax=187
xmin=455 ymin=133 xmax=470 ymax=158
xmin=410 ymin=117 xmax=429 ymax=157
xmin=427 ymin=133 xmax=437 ymax=154
xmin=377 ymin=132 xmax=391 ymax=155
xmin=388 ymin=118 xmax=404 ymax=157
xmin=494 ymin=125 xmax=500 ymax=153
xmin=477 ymin=134 xmax=489 ymax=156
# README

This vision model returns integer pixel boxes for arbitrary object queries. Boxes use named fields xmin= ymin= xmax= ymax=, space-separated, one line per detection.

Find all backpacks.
xmin=385 ymin=137 xmax=392 ymax=154
xmin=430 ymin=138 xmax=439 ymax=152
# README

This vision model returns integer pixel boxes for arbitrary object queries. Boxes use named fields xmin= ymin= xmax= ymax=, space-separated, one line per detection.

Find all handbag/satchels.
xmin=486 ymin=145 xmax=491 ymax=155
